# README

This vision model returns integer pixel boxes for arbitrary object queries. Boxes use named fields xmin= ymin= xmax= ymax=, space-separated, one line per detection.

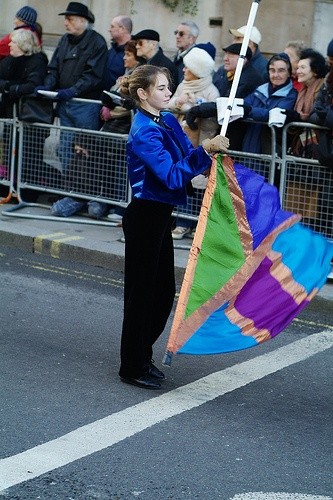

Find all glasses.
xmin=174 ymin=30 xmax=191 ymax=38
xmin=133 ymin=40 xmax=152 ymax=46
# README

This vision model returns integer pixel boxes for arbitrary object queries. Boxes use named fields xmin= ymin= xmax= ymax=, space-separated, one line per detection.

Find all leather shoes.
xmin=119 ymin=369 xmax=162 ymax=390
xmin=146 ymin=358 xmax=166 ymax=381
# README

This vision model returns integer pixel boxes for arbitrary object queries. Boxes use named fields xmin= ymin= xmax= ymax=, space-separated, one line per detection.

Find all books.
xmin=103 ymin=90 xmax=126 ymax=107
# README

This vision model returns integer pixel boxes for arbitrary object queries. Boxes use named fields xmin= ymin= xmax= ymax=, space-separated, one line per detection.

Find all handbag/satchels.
xmin=297 ymin=143 xmax=322 ymax=190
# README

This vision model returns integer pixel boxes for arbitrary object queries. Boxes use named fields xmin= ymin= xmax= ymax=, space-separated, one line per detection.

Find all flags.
xmin=166 ymin=152 xmax=333 ymax=354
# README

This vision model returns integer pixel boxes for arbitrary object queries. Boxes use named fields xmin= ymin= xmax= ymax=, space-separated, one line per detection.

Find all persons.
xmin=285 ymin=40 xmax=311 ymax=92
xmin=169 ymin=21 xmax=200 ymax=84
xmin=305 ymin=61 xmax=333 ymax=265
xmin=217 ymin=25 xmax=271 ymax=85
xmin=237 ymin=53 xmax=299 ymax=194
xmin=33 ymin=2 xmax=177 ymax=222
xmin=325 ymin=39 xmax=333 ymax=66
xmin=185 ymin=43 xmax=263 ymax=176
xmin=1 ymin=6 xmax=43 ymax=56
xmin=189 ymin=42 xmax=224 ymax=83
xmin=168 ymin=47 xmax=221 ymax=239
xmin=0 ymin=28 xmax=54 ymax=205
xmin=119 ymin=65 xmax=230 ymax=390
xmin=280 ymin=48 xmax=330 ymax=234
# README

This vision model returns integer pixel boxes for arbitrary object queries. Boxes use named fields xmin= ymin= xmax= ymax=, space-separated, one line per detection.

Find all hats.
xmin=222 ymin=43 xmax=252 ymax=60
xmin=15 ymin=6 xmax=37 ymax=26
xmin=229 ymin=25 xmax=262 ymax=45
xmin=58 ymin=2 xmax=95 ymax=23
xmin=182 ymin=42 xmax=217 ymax=62
xmin=183 ymin=47 xmax=216 ymax=78
xmin=130 ymin=28 xmax=160 ymax=41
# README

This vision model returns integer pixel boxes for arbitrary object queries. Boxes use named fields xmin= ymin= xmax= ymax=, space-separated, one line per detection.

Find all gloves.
xmin=313 ymin=103 xmax=327 ymax=121
xmin=185 ymin=105 xmax=202 ymax=131
xmin=54 ymin=88 xmax=76 ymax=104
xmin=0 ymin=80 xmax=17 ymax=94
xmin=238 ymin=101 xmax=252 ymax=121
xmin=34 ymin=85 xmax=48 ymax=96
xmin=100 ymin=91 xmax=136 ymax=121
xmin=280 ymin=109 xmax=301 ymax=125
xmin=202 ymin=135 xmax=229 ymax=154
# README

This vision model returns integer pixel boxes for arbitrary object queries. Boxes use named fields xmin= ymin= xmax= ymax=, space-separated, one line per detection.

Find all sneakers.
xmin=171 ymin=225 xmax=191 ymax=240
xmin=107 ymin=212 xmax=123 ymax=220
